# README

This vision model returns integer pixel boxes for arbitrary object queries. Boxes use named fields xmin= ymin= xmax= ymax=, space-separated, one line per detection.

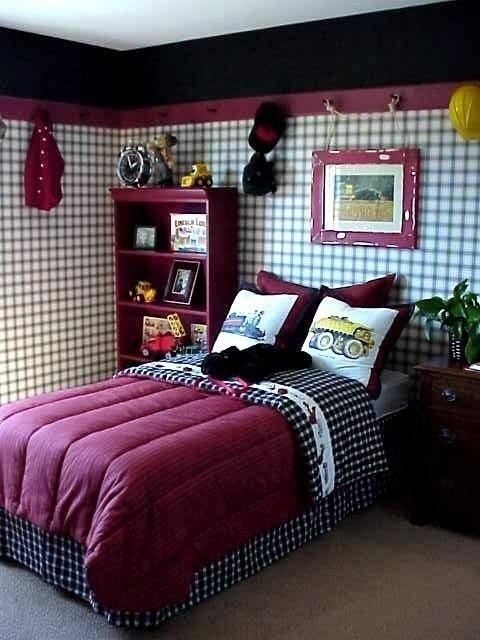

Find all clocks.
xmin=118 ymin=144 xmax=151 ymax=188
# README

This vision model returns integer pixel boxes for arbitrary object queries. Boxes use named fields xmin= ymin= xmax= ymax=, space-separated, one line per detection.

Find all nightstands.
xmin=411 ymin=353 xmax=479 ymax=538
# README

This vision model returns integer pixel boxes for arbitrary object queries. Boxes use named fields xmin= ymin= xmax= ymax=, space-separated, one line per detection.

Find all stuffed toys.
xmin=142 ymin=135 xmax=177 ymax=186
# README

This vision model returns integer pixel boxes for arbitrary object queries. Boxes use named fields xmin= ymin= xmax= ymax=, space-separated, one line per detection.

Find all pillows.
xmin=211 ymin=281 xmax=317 ymax=352
xmin=258 ymin=270 xmax=397 ymax=307
xmin=300 ymin=284 xmax=414 ymax=399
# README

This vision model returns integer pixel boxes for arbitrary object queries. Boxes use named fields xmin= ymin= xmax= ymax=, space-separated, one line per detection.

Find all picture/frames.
xmin=311 ymin=147 xmax=419 ymax=249
xmin=163 ymin=259 xmax=200 ymax=305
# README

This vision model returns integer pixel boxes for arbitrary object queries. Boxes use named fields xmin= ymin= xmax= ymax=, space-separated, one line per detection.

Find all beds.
xmin=0 ymin=270 xmax=413 ymax=628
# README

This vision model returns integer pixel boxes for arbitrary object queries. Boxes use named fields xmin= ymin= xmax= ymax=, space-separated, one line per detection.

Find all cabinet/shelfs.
xmin=110 ymin=187 xmax=237 ymax=371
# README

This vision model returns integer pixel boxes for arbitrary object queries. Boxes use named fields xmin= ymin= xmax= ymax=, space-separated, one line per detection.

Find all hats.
xmin=247 ymin=102 xmax=286 ymax=153
